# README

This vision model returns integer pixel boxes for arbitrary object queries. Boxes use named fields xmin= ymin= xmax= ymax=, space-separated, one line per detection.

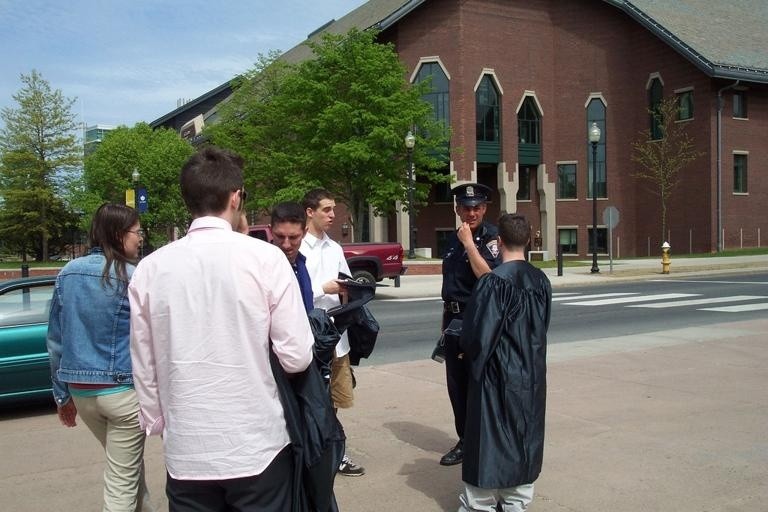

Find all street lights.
xmin=131 ymin=167 xmax=143 ymax=259
xmin=588 ymin=121 xmax=600 ymax=273
xmin=404 ymin=130 xmax=416 ymax=259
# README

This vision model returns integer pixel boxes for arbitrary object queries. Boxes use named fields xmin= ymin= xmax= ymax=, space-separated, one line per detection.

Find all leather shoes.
xmin=441 ymin=441 xmax=464 ymax=466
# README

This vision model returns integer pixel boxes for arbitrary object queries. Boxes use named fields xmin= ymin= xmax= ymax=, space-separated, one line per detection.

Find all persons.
xmin=128 ymin=149 xmax=344 ymax=511
xmin=264 ymin=201 xmax=313 ymax=320
xmin=458 ymin=212 xmax=552 ymax=511
xmin=437 ymin=184 xmax=501 ymax=467
xmin=45 ymin=203 xmax=158 ymax=511
xmin=297 ymin=189 xmax=364 ymax=476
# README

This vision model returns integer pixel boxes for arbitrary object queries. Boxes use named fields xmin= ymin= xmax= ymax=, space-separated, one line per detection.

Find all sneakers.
xmin=337 ymin=455 xmax=365 ymax=477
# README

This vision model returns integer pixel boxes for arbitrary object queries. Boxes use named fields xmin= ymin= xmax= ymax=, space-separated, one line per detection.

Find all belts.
xmin=444 ymin=301 xmax=466 ymax=314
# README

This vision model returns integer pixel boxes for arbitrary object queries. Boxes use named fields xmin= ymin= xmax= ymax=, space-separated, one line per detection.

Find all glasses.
xmin=125 ymin=228 xmax=148 ymax=238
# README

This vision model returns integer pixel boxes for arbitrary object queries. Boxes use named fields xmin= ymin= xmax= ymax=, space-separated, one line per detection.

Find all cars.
xmin=0 ymin=275 xmax=57 ymax=410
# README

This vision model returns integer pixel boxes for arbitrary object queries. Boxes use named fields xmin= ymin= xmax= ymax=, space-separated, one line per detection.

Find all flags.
xmin=126 ymin=189 xmax=136 ymax=209
xmin=138 ymin=188 xmax=148 ymax=213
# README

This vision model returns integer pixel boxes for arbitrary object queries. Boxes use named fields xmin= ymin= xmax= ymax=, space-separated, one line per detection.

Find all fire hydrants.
xmin=660 ymin=241 xmax=671 ymax=274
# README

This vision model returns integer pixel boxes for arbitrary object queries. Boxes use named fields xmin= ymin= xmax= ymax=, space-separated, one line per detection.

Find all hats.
xmin=449 ymin=183 xmax=493 ymax=209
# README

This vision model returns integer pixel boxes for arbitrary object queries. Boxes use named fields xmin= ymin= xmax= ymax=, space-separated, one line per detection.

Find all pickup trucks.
xmin=248 ymin=224 xmax=408 ymax=288
